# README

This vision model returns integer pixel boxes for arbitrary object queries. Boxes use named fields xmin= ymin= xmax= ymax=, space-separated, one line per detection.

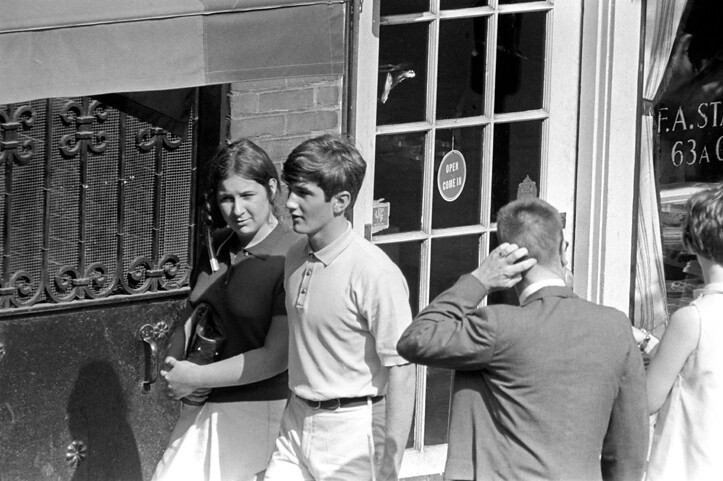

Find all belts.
xmin=296 ymin=394 xmax=383 ymax=409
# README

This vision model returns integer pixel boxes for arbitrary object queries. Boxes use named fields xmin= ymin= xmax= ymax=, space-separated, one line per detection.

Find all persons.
xmin=149 ymin=138 xmax=301 ymax=481
xmin=396 ymin=195 xmax=650 ymax=481
xmin=265 ymin=133 xmax=417 ymax=481
xmin=646 ymin=180 xmax=723 ymax=481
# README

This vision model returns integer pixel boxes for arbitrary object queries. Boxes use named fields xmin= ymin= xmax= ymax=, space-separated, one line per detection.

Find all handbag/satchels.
xmin=182 ymin=302 xmax=224 ymax=407
